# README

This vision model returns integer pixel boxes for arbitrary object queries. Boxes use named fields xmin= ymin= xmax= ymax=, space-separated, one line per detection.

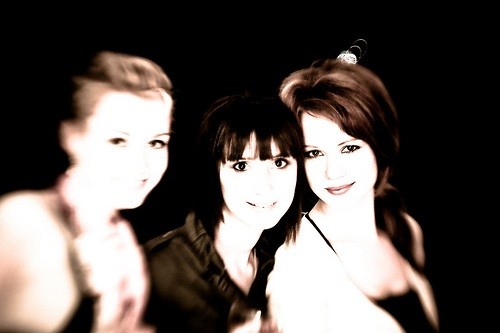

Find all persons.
xmin=277 ymin=57 xmax=440 ymax=333
xmin=0 ymin=51 xmax=175 ymax=333
xmin=139 ymin=87 xmax=305 ymax=333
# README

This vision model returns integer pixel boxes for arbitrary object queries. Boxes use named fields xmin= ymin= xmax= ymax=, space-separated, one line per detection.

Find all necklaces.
xmin=253 ymin=248 xmax=257 ymax=276
